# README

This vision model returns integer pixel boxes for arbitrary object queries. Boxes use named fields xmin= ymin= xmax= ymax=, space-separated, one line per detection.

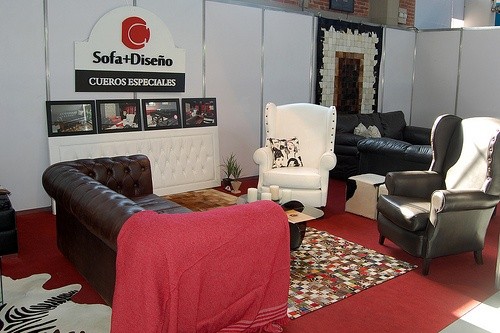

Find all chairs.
xmin=377 ymin=114 xmax=500 ymax=276
xmin=253 ymin=103 xmax=337 ymax=207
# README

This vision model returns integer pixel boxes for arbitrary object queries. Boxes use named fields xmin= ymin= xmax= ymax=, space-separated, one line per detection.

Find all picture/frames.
xmin=142 ymin=98 xmax=182 ymax=131
xmin=96 ymin=99 xmax=143 ymax=134
xmin=182 ymin=98 xmax=217 ymax=128
xmin=46 ymin=100 xmax=97 ymax=137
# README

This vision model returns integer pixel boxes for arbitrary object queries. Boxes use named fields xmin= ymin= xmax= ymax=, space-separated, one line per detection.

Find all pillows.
xmin=335 ymin=111 xmax=409 ymax=141
xmin=267 ymin=137 xmax=303 ymax=169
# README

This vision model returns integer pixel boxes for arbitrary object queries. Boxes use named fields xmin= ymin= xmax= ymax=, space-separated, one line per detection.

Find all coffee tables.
xmin=237 ymin=194 xmax=325 ymax=250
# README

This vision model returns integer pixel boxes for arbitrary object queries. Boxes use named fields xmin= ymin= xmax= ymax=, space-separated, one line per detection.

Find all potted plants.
xmin=220 ymin=152 xmax=242 ymax=194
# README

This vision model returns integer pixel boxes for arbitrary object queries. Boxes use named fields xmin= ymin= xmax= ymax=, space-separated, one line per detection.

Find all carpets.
xmin=161 ymin=189 xmax=419 ymax=319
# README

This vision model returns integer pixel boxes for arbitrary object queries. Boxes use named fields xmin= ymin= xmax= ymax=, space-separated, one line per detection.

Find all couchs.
xmin=330 ymin=111 xmax=432 ymax=183
xmin=43 ymin=155 xmax=290 ymax=333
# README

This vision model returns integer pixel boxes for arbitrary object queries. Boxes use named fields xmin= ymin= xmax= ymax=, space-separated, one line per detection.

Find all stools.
xmin=345 ymin=174 xmax=388 ymax=220
